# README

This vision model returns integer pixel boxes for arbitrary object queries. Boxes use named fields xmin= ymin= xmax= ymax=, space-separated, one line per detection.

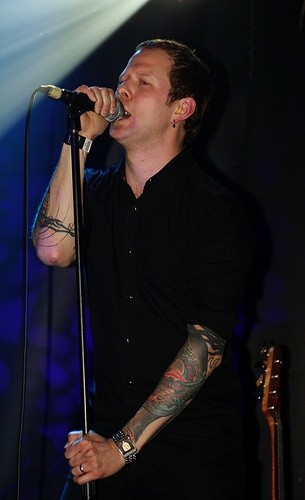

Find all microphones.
xmin=39 ymin=85 xmax=125 ymax=122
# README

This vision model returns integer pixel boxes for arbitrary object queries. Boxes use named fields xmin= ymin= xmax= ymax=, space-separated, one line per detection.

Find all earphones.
xmin=176 ymin=107 xmax=183 ymax=114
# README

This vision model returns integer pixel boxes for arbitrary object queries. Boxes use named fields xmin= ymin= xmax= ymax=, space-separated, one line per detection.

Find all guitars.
xmin=256 ymin=345 xmax=285 ymax=500
